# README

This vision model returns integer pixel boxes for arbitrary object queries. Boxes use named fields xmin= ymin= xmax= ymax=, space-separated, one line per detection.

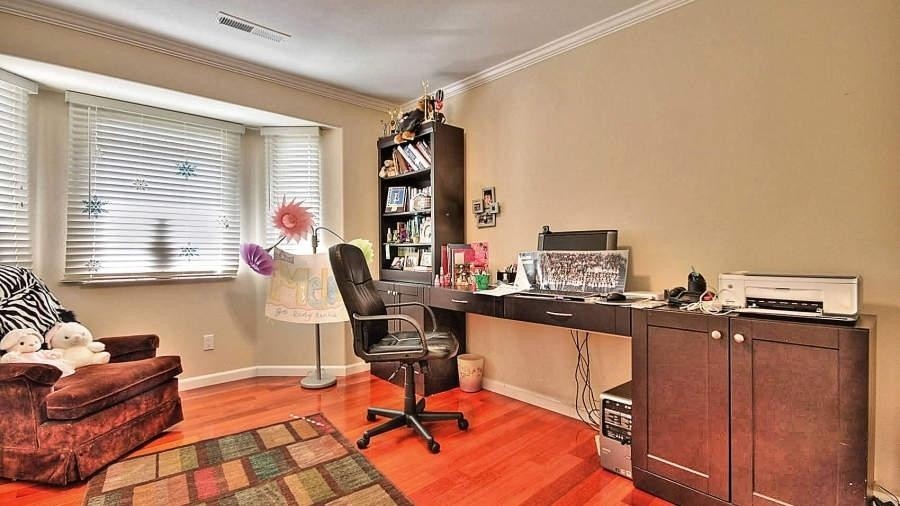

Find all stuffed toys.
xmin=378 ymin=160 xmax=395 ymax=177
xmin=43 ymin=323 xmax=110 ymax=370
xmin=394 ymin=96 xmax=431 ymax=144
xmin=1 ymin=328 xmax=76 ymax=376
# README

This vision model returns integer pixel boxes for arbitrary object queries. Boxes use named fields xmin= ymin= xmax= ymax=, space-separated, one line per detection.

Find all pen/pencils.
xmin=506 ymin=264 xmax=517 ymax=273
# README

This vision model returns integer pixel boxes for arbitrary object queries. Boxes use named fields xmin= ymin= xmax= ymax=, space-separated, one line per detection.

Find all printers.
xmin=718 ymin=271 xmax=859 ymax=322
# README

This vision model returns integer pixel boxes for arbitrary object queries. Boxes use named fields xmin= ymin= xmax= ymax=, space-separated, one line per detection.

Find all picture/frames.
xmin=481 ymin=187 xmax=494 ymax=208
xmin=477 ymin=210 xmax=495 ymax=227
xmin=420 ymin=253 xmax=432 ymax=266
xmin=390 ymin=256 xmax=405 ymax=269
xmin=490 ymin=202 xmax=499 ymax=213
xmin=472 ymin=200 xmax=483 ymax=213
xmin=404 ymin=253 xmax=418 ymax=270
xmin=386 ymin=187 xmax=405 ymax=212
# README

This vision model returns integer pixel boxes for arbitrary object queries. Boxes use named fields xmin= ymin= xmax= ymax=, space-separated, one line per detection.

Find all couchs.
xmin=0 ymin=264 xmax=183 ymax=486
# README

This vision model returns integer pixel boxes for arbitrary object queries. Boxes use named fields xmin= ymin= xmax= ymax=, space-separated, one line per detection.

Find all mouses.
xmin=606 ymin=293 xmax=626 ymax=302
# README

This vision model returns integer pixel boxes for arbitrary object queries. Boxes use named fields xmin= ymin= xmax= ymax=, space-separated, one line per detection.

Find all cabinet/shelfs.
xmin=371 ymin=123 xmax=464 ymax=396
xmin=632 ymin=302 xmax=878 ymax=506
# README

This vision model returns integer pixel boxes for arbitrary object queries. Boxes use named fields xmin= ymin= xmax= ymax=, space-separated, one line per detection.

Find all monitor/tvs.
xmin=538 ymin=230 xmax=618 ymax=250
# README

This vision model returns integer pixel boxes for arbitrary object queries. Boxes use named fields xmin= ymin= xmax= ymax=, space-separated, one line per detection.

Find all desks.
xmin=432 ymin=278 xmax=665 ymax=339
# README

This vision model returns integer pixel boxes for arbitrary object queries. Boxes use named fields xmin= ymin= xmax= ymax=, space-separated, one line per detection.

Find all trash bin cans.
xmin=457 ymin=353 xmax=485 ymax=393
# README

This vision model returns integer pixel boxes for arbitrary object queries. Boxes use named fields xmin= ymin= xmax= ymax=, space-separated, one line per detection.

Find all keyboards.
xmin=518 ymin=288 xmax=602 ymax=302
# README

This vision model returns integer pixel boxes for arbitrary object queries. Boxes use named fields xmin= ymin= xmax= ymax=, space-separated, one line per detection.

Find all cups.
xmin=474 ymin=273 xmax=490 ymax=289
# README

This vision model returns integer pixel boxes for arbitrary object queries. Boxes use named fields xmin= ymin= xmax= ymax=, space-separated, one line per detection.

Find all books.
xmin=447 ymin=243 xmax=475 ymax=287
xmin=393 ymin=140 xmax=432 ymax=174
xmin=385 ymin=186 xmax=431 ymax=212
xmin=406 ymin=216 xmax=431 ymax=243
xmin=398 ymin=246 xmax=433 ymax=258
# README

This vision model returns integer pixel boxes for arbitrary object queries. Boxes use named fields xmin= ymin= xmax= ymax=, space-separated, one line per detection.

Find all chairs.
xmin=329 ymin=244 xmax=468 ymax=453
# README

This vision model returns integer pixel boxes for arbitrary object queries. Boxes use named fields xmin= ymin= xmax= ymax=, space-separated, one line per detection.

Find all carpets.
xmin=83 ymin=415 xmax=414 ymax=506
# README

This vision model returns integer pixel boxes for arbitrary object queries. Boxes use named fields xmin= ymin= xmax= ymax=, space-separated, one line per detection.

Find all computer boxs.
xmin=600 ymin=380 xmax=633 ymax=480
